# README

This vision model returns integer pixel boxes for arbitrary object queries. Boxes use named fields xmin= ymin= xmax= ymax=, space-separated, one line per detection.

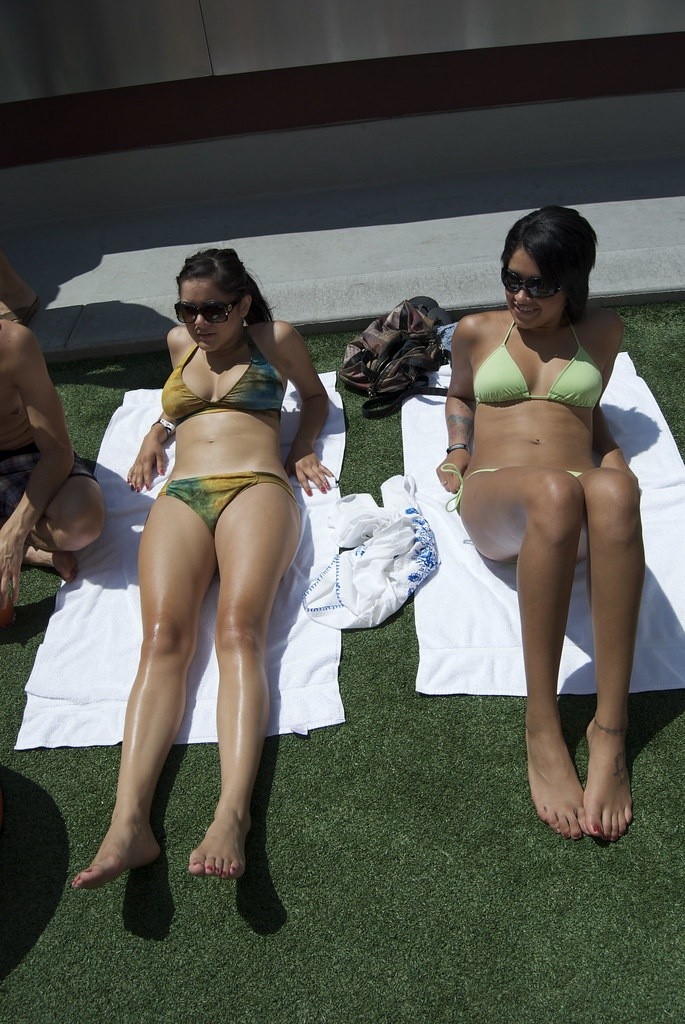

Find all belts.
xmin=362 ymin=387 xmax=448 ymax=420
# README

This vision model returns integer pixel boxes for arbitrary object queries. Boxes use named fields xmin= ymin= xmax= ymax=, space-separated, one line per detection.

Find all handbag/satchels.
xmin=339 ymin=297 xmax=452 ymax=395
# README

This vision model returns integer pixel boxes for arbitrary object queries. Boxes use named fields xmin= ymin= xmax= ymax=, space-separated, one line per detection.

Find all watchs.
xmin=152 ymin=418 xmax=175 ymax=438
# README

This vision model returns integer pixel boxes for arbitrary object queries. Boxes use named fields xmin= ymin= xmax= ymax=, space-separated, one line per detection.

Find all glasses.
xmin=174 ymin=300 xmax=239 ymax=323
xmin=501 ymin=266 xmax=567 ymax=300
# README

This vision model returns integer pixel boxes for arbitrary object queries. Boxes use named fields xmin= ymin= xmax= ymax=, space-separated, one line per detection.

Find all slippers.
xmin=0 ymin=295 xmax=41 ymax=327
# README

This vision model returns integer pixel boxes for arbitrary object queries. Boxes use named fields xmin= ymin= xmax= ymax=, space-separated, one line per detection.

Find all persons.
xmin=0 ymin=247 xmax=105 ymax=631
xmin=71 ymin=248 xmax=332 ymax=892
xmin=437 ymin=204 xmax=648 ymax=843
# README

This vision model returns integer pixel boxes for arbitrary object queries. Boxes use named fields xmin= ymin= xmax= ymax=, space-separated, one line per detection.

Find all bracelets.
xmin=446 ymin=443 xmax=470 ymax=455
